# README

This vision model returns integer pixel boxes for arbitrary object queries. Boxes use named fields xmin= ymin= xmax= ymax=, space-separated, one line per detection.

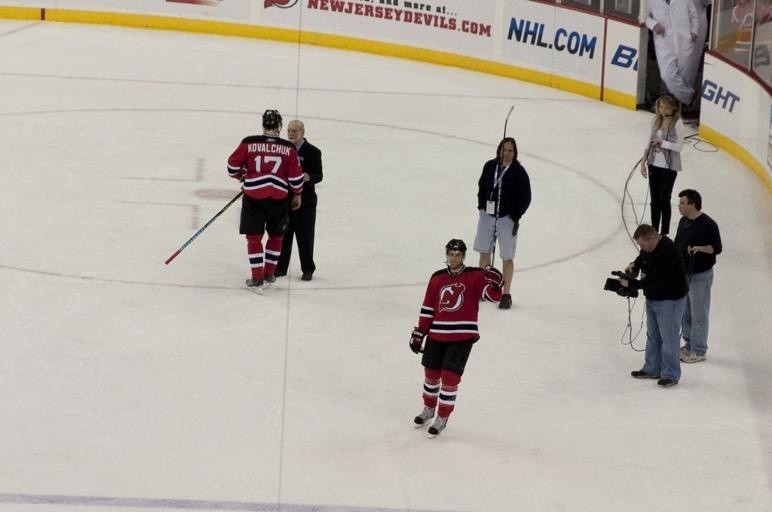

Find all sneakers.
xmin=631 ymin=369 xmax=661 ymax=379
xmin=677 ymin=345 xmax=688 ymax=359
xmin=689 ymin=89 xmax=698 ymax=113
xmin=681 ymin=101 xmax=698 ymax=124
xmin=246 ymin=267 xmax=316 ymax=287
xmin=428 ymin=416 xmax=448 ymax=435
xmin=680 ymin=352 xmax=706 ymax=363
xmin=498 ymin=293 xmax=512 ymax=309
xmin=657 ymin=378 xmax=674 ymax=386
xmin=414 ymin=404 xmax=435 ymax=424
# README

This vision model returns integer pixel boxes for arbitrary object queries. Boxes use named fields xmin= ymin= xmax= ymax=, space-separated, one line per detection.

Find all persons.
xmin=272 ymin=120 xmax=323 ymax=281
xmin=637 ymin=0 xmax=700 ymax=115
xmin=640 ymin=92 xmax=685 ymax=235
xmin=618 ymin=224 xmax=690 ymax=385
xmin=409 ymin=238 xmax=505 ymax=438
xmin=729 ymin=0 xmax=772 ymax=72
xmin=688 ymin=0 xmax=712 ymax=89
xmin=226 ymin=109 xmax=305 ymax=296
xmin=673 ymin=188 xmax=723 ymax=363
xmin=473 ymin=137 xmax=532 ymax=309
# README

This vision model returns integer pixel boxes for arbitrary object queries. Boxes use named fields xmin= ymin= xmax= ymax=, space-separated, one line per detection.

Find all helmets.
xmin=445 ymin=238 xmax=468 ymax=256
xmin=261 ymin=108 xmax=283 ymax=131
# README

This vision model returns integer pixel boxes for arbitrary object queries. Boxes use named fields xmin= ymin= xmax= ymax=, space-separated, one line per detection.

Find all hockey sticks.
xmin=488 ymin=105 xmax=515 ymax=267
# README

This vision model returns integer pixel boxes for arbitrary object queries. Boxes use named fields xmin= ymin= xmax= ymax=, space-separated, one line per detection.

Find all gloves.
xmin=484 ymin=264 xmax=505 ymax=292
xmin=408 ymin=326 xmax=425 ymax=354
